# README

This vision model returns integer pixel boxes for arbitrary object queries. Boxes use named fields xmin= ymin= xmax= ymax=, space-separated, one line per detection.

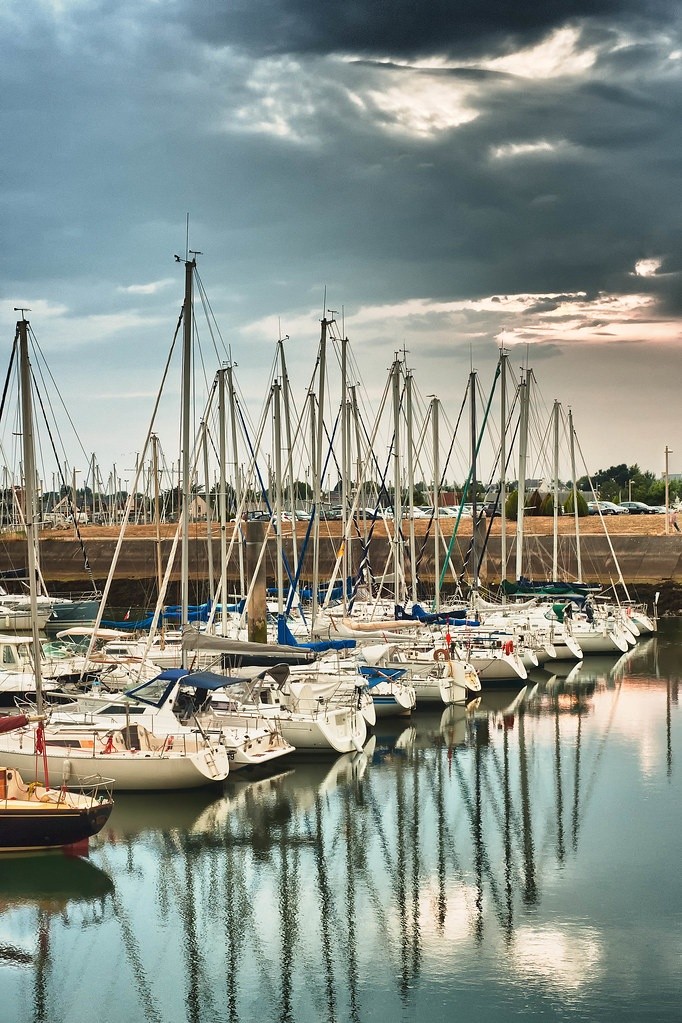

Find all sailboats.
xmin=0 ymin=305 xmax=116 ymax=851
xmin=0 ymin=209 xmax=680 ymax=792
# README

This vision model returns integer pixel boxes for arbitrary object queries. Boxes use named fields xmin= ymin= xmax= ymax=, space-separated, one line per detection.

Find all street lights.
xmin=664 ymin=444 xmax=674 ymax=534
xmin=628 ymin=478 xmax=636 ymax=502
xmin=595 ymin=482 xmax=600 ymax=498
xmin=125 ymin=480 xmax=129 ymax=494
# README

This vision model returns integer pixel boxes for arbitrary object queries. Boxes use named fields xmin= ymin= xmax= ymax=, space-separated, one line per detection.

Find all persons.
xmin=670 ymin=511 xmax=681 ymax=532
